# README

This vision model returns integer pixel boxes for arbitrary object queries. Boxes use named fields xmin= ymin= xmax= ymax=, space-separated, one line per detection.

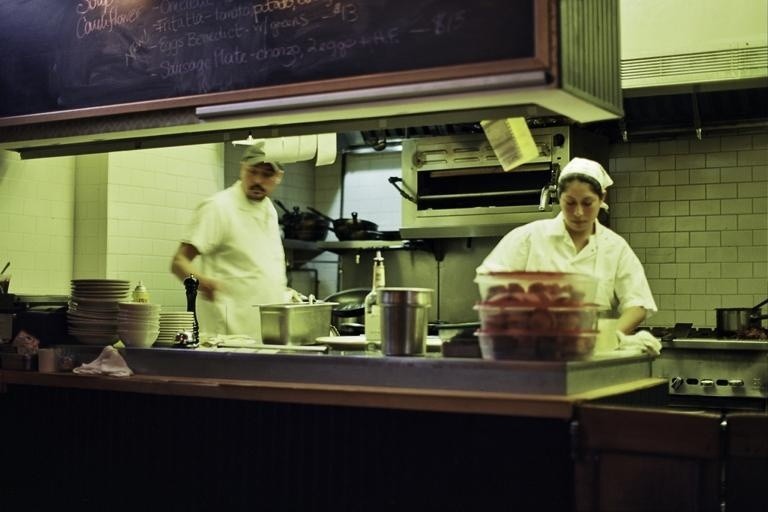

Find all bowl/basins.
xmin=469 ymin=265 xmax=607 ymax=360
xmin=115 ymin=300 xmax=163 ymax=348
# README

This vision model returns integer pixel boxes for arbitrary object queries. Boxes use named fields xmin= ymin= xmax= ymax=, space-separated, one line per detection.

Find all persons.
xmin=170 ymin=139 xmax=289 ymax=338
xmin=476 ymin=157 xmax=660 ymax=353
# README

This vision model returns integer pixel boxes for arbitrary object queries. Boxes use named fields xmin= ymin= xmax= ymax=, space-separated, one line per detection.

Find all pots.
xmin=713 ymin=297 xmax=766 ymax=336
xmin=272 ymin=197 xmax=384 ymax=240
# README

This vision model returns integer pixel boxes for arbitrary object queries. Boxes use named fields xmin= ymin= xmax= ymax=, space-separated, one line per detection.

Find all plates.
xmin=155 ymin=311 xmax=194 ymax=346
xmin=65 ymin=277 xmax=130 ymax=344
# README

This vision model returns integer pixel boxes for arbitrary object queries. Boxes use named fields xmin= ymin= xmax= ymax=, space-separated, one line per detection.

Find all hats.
xmin=241 ymin=140 xmax=285 ymax=170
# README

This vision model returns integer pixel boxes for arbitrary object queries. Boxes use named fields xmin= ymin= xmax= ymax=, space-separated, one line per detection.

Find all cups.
xmin=374 ymin=285 xmax=435 ymax=357
xmin=0 ymin=272 xmax=12 ymax=293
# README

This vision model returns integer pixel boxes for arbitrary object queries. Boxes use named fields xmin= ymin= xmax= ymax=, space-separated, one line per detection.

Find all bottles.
xmin=364 ymin=249 xmax=388 ymax=347
xmin=181 ymin=272 xmax=201 ymax=346
xmin=130 ymin=279 xmax=150 ymax=302
